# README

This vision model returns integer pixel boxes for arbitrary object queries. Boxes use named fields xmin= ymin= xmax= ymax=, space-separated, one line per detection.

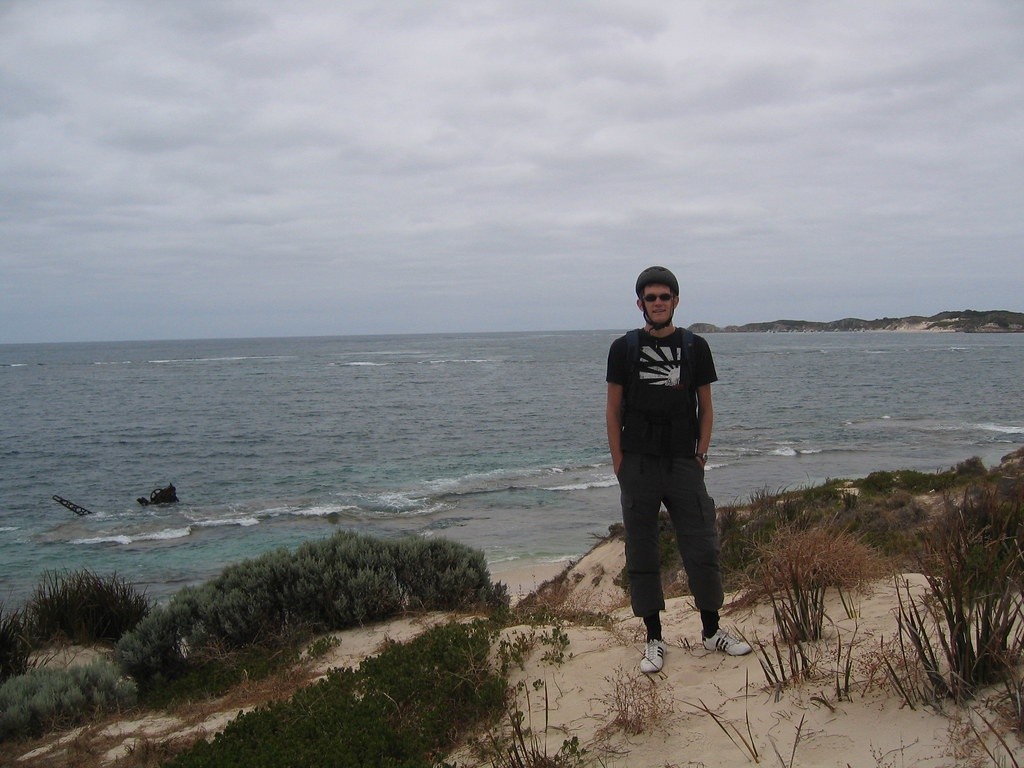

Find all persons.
xmin=605 ymin=267 xmax=753 ymax=672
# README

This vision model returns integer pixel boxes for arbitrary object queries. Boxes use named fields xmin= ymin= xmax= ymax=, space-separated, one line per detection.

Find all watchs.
xmin=694 ymin=452 xmax=709 ymax=462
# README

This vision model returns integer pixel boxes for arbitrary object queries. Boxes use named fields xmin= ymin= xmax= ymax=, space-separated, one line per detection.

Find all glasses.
xmin=642 ymin=294 xmax=675 ymax=302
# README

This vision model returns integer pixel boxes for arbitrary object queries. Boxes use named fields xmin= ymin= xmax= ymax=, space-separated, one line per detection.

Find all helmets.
xmin=636 ymin=266 xmax=679 ymax=296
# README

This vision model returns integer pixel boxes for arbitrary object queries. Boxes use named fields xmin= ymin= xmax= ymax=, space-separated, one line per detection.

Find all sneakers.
xmin=640 ymin=639 xmax=666 ymax=672
xmin=701 ymin=628 xmax=752 ymax=656
